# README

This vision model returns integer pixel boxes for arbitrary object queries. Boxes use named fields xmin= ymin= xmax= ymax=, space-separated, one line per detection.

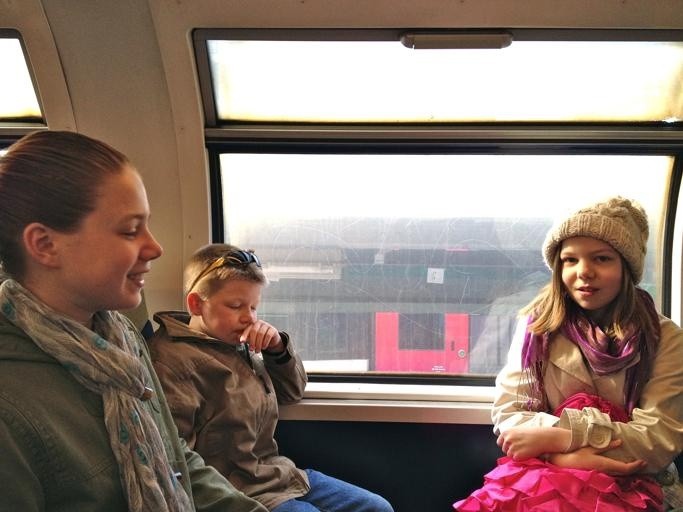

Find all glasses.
xmin=186 ymin=249 xmax=262 ymax=294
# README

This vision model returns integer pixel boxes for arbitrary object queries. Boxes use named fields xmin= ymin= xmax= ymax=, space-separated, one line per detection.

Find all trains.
xmin=222 ymin=214 xmax=654 ymax=371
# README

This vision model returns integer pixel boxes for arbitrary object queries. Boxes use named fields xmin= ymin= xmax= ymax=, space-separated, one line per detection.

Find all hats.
xmin=541 ymin=196 xmax=650 ymax=284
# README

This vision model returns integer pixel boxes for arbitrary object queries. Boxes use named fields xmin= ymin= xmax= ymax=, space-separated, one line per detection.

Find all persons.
xmin=138 ymin=242 xmax=395 ymax=512
xmin=0 ymin=127 xmax=271 ymax=512
xmin=451 ymin=195 xmax=681 ymax=512
